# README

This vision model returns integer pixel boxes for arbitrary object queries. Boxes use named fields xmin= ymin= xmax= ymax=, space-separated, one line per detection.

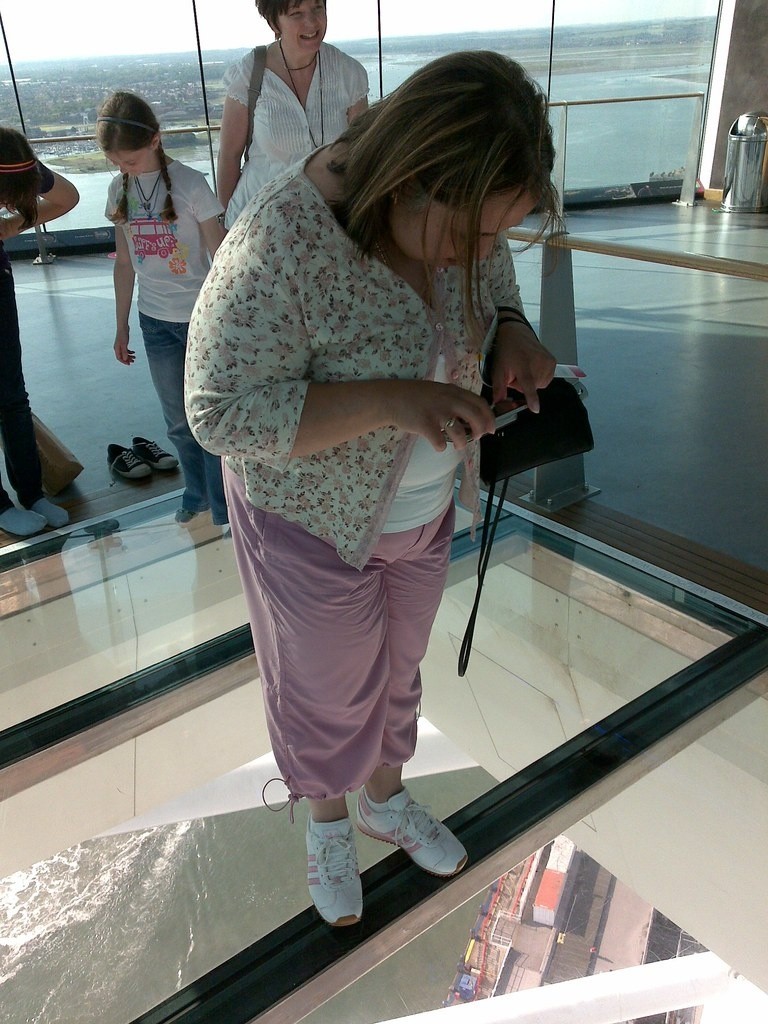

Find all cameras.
xmin=442 ymin=398 xmax=527 ymax=444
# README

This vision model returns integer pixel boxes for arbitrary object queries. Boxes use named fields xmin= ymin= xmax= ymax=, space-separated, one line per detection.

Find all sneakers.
xmin=221 ymin=526 xmax=232 ymax=541
xmin=105 ymin=443 xmax=152 ymax=478
xmin=355 ymin=785 xmax=468 ymax=878
xmin=174 ymin=507 xmax=211 ymax=524
xmin=131 ymin=436 xmax=180 ymax=470
xmin=305 ymin=809 xmax=364 ymax=927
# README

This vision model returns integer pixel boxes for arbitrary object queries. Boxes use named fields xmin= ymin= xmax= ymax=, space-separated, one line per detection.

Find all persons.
xmin=0 ymin=125 xmax=81 ymax=536
xmin=180 ymin=46 xmax=558 ymax=930
xmin=99 ymin=88 xmax=233 ymax=525
xmin=215 ymin=0 xmax=370 ymax=233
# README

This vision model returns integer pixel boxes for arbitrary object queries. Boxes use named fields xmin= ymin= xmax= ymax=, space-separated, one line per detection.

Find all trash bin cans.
xmin=720 ymin=111 xmax=768 ymax=213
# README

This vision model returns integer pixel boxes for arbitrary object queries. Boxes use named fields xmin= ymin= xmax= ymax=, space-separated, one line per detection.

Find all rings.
xmin=444 ymin=416 xmax=456 ymax=428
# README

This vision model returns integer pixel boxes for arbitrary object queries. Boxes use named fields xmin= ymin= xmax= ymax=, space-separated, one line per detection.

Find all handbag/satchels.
xmin=30 ymin=410 xmax=84 ymax=495
xmin=478 ymin=306 xmax=594 ymax=485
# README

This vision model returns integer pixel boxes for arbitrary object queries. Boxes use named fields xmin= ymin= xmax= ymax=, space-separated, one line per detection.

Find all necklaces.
xmin=279 ymin=37 xmax=324 ymax=149
xmin=372 ymin=232 xmax=430 ymax=301
xmin=135 ymin=168 xmax=163 ymax=221
